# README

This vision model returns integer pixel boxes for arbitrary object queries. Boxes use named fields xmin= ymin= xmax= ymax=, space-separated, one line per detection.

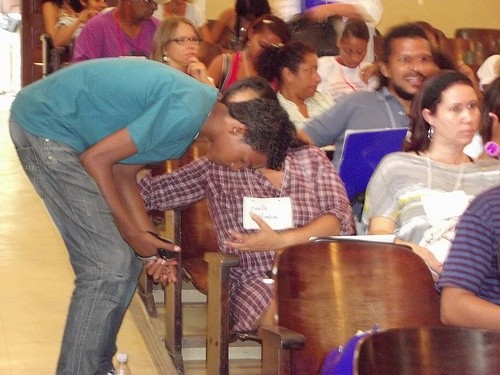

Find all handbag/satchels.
xmin=287 ymin=13 xmax=339 ymax=56
xmin=0 ymin=15 xmax=19 ymax=32
xmin=418 ymin=218 xmax=460 ymax=277
xmin=317 ymin=322 xmax=381 ymax=375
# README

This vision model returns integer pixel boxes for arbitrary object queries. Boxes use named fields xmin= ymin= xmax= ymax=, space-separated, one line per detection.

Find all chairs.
xmin=40 ymin=21 xmax=500 ymax=375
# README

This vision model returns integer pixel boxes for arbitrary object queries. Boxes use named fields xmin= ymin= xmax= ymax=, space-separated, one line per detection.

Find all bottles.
xmin=112 ymin=353 xmax=132 ymax=375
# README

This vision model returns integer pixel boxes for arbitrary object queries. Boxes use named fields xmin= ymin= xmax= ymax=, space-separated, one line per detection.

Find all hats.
xmin=476 ymin=54 xmax=500 ymax=91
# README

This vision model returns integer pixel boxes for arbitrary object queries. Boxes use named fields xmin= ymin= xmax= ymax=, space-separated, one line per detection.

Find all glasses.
xmin=258 ymin=19 xmax=277 ymax=24
xmin=169 ymin=36 xmax=201 ymax=45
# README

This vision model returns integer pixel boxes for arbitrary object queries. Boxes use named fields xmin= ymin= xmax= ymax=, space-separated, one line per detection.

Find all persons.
xmin=361 ymin=72 xmax=500 ymax=278
xmin=9 ymin=58 xmax=297 ymax=375
xmin=41 ymin=0 xmax=499 ymax=174
xmin=435 ymin=184 xmax=499 ymax=329
xmin=137 ymin=77 xmax=357 ymax=337
